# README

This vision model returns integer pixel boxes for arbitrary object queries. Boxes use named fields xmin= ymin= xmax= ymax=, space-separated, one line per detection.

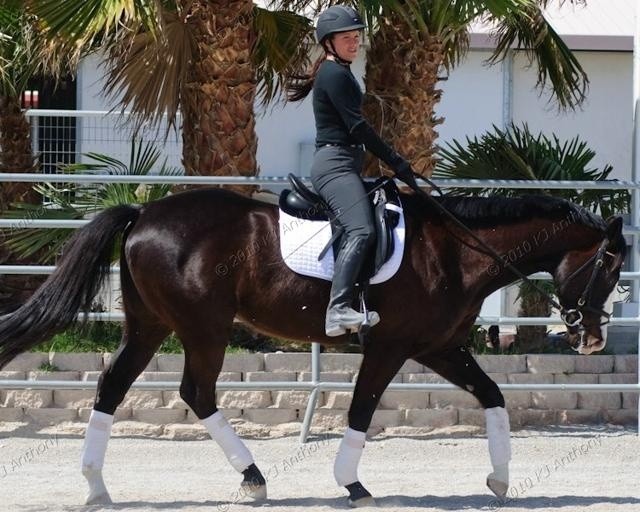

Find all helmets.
xmin=316 ymin=4 xmax=366 ymax=42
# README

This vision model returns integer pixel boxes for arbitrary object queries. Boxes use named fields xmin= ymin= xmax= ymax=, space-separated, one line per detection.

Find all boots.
xmin=325 ymin=234 xmax=378 ymax=337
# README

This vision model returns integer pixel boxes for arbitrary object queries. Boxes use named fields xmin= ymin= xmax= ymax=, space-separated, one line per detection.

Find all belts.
xmin=325 ymin=143 xmax=367 ymax=152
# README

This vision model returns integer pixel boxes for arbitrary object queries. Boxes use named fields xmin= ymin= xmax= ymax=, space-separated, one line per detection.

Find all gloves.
xmin=393 ymin=156 xmax=413 ymax=179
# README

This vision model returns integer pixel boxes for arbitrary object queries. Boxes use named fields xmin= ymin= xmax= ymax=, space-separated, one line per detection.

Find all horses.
xmin=0 ymin=188 xmax=627 ymax=507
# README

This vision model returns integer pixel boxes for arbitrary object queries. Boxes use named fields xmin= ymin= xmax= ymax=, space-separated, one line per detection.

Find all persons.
xmin=309 ymin=4 xmax=416 ymax=338
